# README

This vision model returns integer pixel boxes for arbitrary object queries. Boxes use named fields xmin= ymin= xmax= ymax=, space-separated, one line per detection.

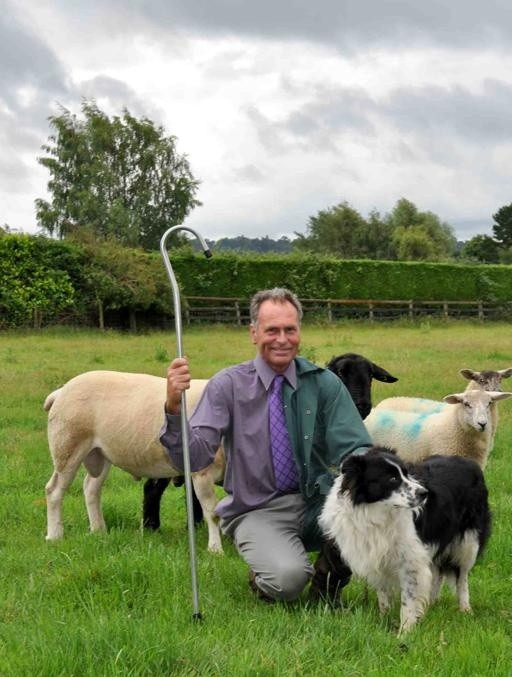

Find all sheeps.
xmin=375 ymin=366 xmax=512 ymax=413
xmin=140 ymin=351 xmax=401 ymax=544
xmin=360 ymin=387 xmax=512 ymax=473
xmin=39 ymin=367 xmax=228 ymax=557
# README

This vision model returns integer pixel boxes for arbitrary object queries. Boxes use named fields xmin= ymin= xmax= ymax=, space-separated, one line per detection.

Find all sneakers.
xmin=248 ymin=567 xmax=275 ymax=604
xmin=309 ymin=586 xmax=347 ymax=613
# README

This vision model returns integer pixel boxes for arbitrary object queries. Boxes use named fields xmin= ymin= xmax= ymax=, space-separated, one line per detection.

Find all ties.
xmin=268 ymin=375 xmax=299 ymax=493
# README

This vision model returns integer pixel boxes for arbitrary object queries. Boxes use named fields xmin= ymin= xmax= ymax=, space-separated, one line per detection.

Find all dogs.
xmin=316 ymin=443 xmax=493 ymax=643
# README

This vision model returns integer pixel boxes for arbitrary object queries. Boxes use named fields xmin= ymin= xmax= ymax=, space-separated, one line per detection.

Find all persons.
xmin=158 ymin=287 xmax=373 ymax=603
